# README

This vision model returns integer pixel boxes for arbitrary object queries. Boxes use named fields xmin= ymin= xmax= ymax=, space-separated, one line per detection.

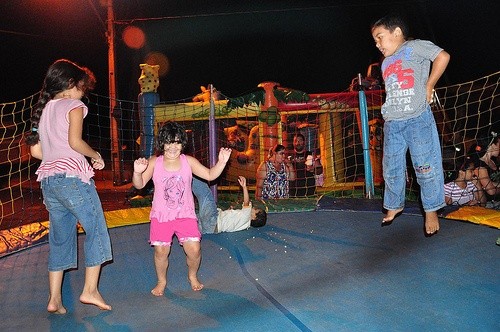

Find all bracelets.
xmin=92 ymin=151 xmax=102 ymax=162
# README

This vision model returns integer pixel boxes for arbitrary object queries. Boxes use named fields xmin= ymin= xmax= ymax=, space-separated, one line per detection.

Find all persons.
xmin=444 ymin=131 xmax=500 ymax=207
xmin=192 ymin=176 xmax=267 ymax=234
xmin=285 ymin=134 xmax=323 ymax=196
xmin=132 ymin=119 xmax=232 ymax=297
xmin=26 ymin=59 xmax=112 ymax=314
xmin=255 ymin=144 xmax=297 ymax=201
xmin=371 ymin=16 xmax=450 ymax=234
